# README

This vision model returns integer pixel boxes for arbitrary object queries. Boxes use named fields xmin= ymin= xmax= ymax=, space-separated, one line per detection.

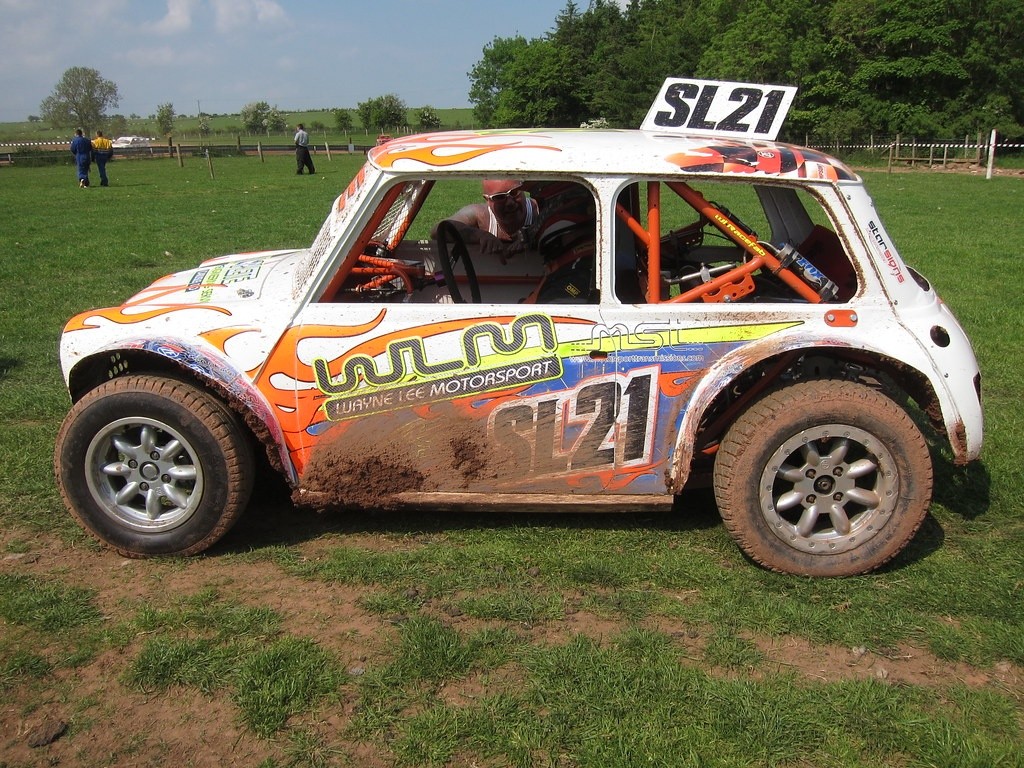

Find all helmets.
xmin=536 ymin=208 xmax=597 ymax=272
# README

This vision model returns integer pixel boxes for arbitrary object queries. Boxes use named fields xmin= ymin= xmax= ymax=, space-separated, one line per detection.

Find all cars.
xmin=376 ymin=135 xmax=392 ymax=146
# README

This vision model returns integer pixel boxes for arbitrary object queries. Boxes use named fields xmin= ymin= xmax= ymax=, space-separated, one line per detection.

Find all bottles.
xmin=723 ymin=295 xmax=735 ymax=303
xmin=700 ymin=262 xmax=710 ymax=283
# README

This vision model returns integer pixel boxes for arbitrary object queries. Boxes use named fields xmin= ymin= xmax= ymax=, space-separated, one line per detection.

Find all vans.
xmin=112 ymin=136 xmax=140 ymax=148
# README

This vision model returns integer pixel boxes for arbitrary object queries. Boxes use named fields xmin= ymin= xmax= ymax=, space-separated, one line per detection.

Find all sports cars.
xmin=55 ymin=126 xmax=982 ymax=583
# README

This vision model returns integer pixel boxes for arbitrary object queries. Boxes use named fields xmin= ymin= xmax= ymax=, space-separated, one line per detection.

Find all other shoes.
xmin=80 ymin=179 xmax=85 ymax=187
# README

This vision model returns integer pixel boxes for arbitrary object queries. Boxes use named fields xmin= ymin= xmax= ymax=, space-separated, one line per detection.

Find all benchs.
xmin=701 ymin=224 xmax=858 ymax=303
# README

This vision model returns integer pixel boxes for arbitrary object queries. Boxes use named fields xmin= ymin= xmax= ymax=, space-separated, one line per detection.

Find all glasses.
xmin=486 ymin=185 xmax=522 ymax=203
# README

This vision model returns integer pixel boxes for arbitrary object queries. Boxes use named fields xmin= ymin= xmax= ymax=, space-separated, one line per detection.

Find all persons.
xmin=71 ymin=129 xmax=112 ymax=189
xmin=293 ymin=123 xmax=316 ymax=175
xmin=431 ymin=180 xmax=539 ymax=255
xmin=517 ymin=210 xmax=594 ymax=304
